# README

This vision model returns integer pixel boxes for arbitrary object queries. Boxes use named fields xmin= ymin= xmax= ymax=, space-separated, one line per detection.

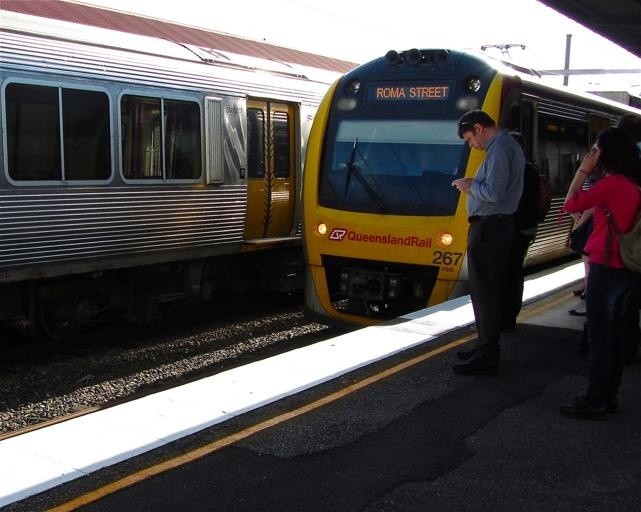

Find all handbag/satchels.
xmin=525 ymin=158 xmax=553 ymax=224
xmin=620 ymin=214 xmax=641 ymax=272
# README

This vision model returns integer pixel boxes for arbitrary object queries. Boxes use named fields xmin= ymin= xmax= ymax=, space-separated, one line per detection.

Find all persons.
xmin=449 ymin=111 xmax=526 ymax=342
xmin=506 ymin=131 xmax=553 ymax=334
xmin=558 ymin=114 xmax=640 ymax=419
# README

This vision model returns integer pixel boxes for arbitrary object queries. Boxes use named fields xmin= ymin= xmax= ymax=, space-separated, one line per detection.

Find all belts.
xmin=467 ymin=215 xmax=502 ymax=222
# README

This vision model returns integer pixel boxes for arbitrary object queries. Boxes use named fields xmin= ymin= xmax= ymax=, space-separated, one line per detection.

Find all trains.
xmin=289 ymin=44 xmax=641 ymax=328
xmin=0 ymin=1 xmax=361 ymax=284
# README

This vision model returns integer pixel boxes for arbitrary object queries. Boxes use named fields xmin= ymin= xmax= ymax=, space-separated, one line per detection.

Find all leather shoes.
xmin=560 ymin=392 xmax=618 ymax=419
xmin=453 ymin=340 xmax=500 ymax=373
